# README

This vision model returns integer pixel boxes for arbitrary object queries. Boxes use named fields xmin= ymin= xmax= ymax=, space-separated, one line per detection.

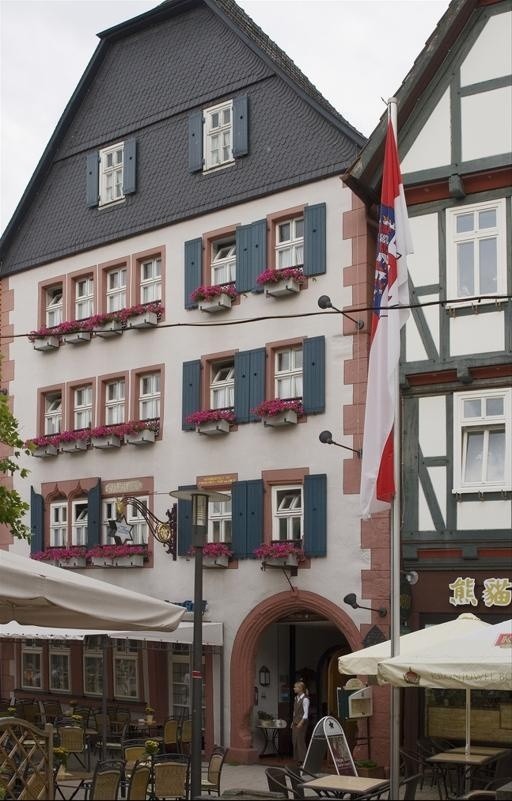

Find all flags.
xmin=358 ymin=109 xmax=414 ymax=522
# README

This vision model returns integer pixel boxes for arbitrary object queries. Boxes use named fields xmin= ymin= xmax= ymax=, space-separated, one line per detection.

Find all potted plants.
xmin=354 ymin=759 xmax=385 ymax=777
xmin=258 ymin=710 xmax=273 ymax=727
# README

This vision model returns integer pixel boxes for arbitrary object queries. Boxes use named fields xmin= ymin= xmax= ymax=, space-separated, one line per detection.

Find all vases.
xmin=33 ymin=336 xmax=59 ymax=351
xmin=59 ymin=442 xmax=87 ymax=454
xmin=263 ymin=276 xmax=300 ymax=297
xmin=261 ymin=410 xmax=298 ymax=428
xmin=40 ymin=555 xmax=144 ymax=568
xmin=261 ymin=552 xmax=298 ymax=568
xmin=198 ymin=295 xmax=232 ymax=313
xmin=123 ymin=429 xmax=155 ymax=446
xmin=31 ymin=445 xmax=57 ymax=458
xmin=196 ymin=419 xmax=230 ymax=435
xmin=202 ymin=556 xmax=229 ymax=569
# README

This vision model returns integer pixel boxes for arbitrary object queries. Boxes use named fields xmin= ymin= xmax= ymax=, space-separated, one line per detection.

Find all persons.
xmin=290 ymin=680 xmax=310 ymax=768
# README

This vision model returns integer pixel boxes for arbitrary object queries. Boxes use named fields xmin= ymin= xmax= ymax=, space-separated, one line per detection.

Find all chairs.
xmin=0 ymin=696 xmax=229 ymax=801
xmin=399 ymin=736 xmax=459 ymax=801
xmin=284 ymin=763 xmax=336 ymax=800
xmin=265 ymin=768 xmax=322 ymax=801
xmin=353 ymin=772 xmax=423 ymax=800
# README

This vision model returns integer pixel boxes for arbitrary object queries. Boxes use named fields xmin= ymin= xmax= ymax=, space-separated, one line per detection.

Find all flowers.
xmin=185 ymin=410 xmax=235 ymax=425
xmin=253 ymin=543 xmax=306 ymax=562
xmin=22 ymin=415 xmax=145 ymax=449
xmin=186 ymin=543 xmax=234 ymax=559
xmin=256 ymin=267 xmax=307 ymax=285
xmin=28 ymin=545 xmax=146 ymax=561
xmin=25 ymin=302 xmax=164 ymax=344
xmin=190 ymin=284 xmax=240 ymax=304
xmin=250 ymin=399 xmax=304 ymax=417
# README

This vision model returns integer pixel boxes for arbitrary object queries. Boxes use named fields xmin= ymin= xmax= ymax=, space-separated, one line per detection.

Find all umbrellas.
xmin=378 ymin=614 xmax=512 ymax=688
xmin=338 ymin=612 xmax=495 ymax=794
xmin=1 ymin=546 xmax=187 ymax=632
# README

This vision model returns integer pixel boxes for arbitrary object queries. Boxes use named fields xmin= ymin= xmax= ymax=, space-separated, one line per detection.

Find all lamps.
xmin=343 ymin=593 xmax=387 ymax=617
xmin=318 ymin=295 xmax=364 ymax=329
xmin=259 ymin=664 xmax=270 ymax=685
xmin=319 ymin=430 xmax=362 ymax=458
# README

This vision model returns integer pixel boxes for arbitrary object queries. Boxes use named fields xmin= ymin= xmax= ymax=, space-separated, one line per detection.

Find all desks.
xmin=255 ymin=725 xmax=286 ymax=759
xmin=297 ymin=774 xmax=389 ymax=799
xmin=426 ymin=746 xmax=510 ymax=800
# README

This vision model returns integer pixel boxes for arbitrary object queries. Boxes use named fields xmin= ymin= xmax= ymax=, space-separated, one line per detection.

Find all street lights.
xmin=171 ymin=482 xmax=231 ymax=799
